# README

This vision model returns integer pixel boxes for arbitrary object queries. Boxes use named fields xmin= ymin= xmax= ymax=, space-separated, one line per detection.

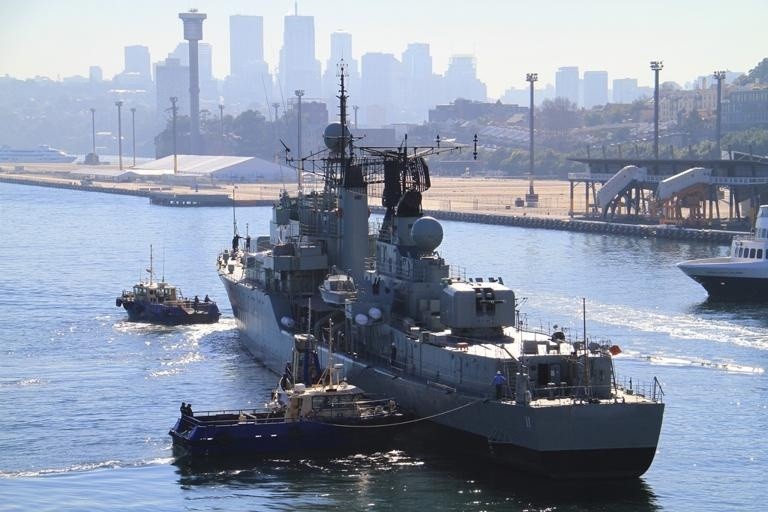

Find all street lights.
xmin=712 ymin=70 xmax=726 ymax=158
xmin=218 ymin=105 xmax=226 ymax=143
xmin=352 ymin=106 xmax=359 ymax=129
xmin=170 ymin=96 xmax=179 ymax=175
xmin=295 ymin=89 xmax=305 ymax=158
xmin=526 ymin=73 xmax=537 ymax=195
xmin=130 ymin=107 xmax=136 ymax=164
xmin=650 ymin=61 xmax=665 ymax=158
xmin=272 ymin=103 xmax=281 ymax=159
xmin=115 ymin=100 xmax=124 ymax=168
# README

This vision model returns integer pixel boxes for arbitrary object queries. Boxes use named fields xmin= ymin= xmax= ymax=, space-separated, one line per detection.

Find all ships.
xmin=215 ymin=57 xmax=664 ymax=479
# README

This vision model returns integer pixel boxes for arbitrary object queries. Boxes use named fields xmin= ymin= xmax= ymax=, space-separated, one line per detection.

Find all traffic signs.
xmin=89 ymin=108 xmax=96 ymax=153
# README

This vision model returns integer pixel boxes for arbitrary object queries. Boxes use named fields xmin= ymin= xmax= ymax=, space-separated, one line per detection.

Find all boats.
xmin=168 ymin=291 xmax=411 ymax=465
xmin=677 ymin=203 xmax=767 ymax=301
xmin=115 ymin=244 xmax=221 ymax=325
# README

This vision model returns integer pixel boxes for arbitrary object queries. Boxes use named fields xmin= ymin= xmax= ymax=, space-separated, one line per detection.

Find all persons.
xmin=194 ymin=295 xmax=199 ymax=303
xmin=205 ymin=294 xmax=211 ymax=302
xmin=180 ymin=402 xmax=187 ymax=415
xmin=244 ymin=234 xmax=252 ymax=253
xmin=184 ymin=403 xmax=193 ymax=416
xmin=232 ymin=235 xmax=237 ymax=251
xmin=489 ymin=369 xmax=506 ymax=400
xmin=236 ymin=233 xmax=243 ymax=250
